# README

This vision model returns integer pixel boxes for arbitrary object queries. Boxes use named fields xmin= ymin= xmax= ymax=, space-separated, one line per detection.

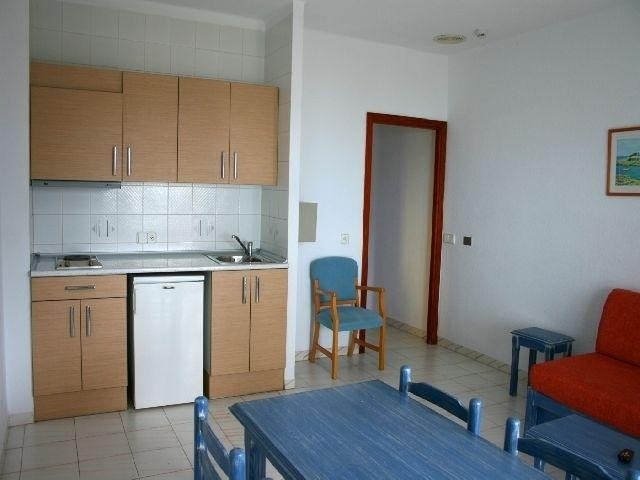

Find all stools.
xmin=509 ymin=327 xmax=575 ymax=397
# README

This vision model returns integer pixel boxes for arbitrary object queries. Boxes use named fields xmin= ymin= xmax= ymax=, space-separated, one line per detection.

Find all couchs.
xmin=525 ymin=288 xmax=640 ymax=434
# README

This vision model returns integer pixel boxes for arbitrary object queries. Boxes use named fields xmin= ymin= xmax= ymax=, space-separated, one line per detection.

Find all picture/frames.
xmin=607 ymin=126 xmax=640 ymax=196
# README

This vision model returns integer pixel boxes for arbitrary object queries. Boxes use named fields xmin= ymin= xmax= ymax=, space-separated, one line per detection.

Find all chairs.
xmin=504 ymin=417 xmax=640 ymax=480
xmin=310 ymin=256 xmax=386 ymax=380
xmin=400 ymin=366 xmax=481 ymax=436
xmin=193 ymin=396 xmax=246 ymax=480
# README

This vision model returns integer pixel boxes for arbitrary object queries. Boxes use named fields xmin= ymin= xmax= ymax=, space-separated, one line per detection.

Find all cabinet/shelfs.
xmin=204 ymin=268 xmax=288 ymax=399
xmin=31 ymin=274 xmax=128 ymax=423
xmin=28 ymin=61 xmax=279 ymax=186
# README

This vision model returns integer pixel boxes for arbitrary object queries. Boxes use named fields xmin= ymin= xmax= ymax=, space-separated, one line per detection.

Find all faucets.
xmin=232 ymin=235 xmax=253 ymax=257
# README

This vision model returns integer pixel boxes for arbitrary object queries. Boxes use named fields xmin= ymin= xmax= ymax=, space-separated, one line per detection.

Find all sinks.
xmin=202 ymin=252 xmax=281 ymax=265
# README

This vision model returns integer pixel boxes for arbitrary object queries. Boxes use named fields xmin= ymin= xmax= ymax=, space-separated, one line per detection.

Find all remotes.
xmin=617 ymin=448 xmax=635 ymax=463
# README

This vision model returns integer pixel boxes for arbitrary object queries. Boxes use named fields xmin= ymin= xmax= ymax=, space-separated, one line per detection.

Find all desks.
xmin=227 ymin=379 xmax=560 ymax=480
